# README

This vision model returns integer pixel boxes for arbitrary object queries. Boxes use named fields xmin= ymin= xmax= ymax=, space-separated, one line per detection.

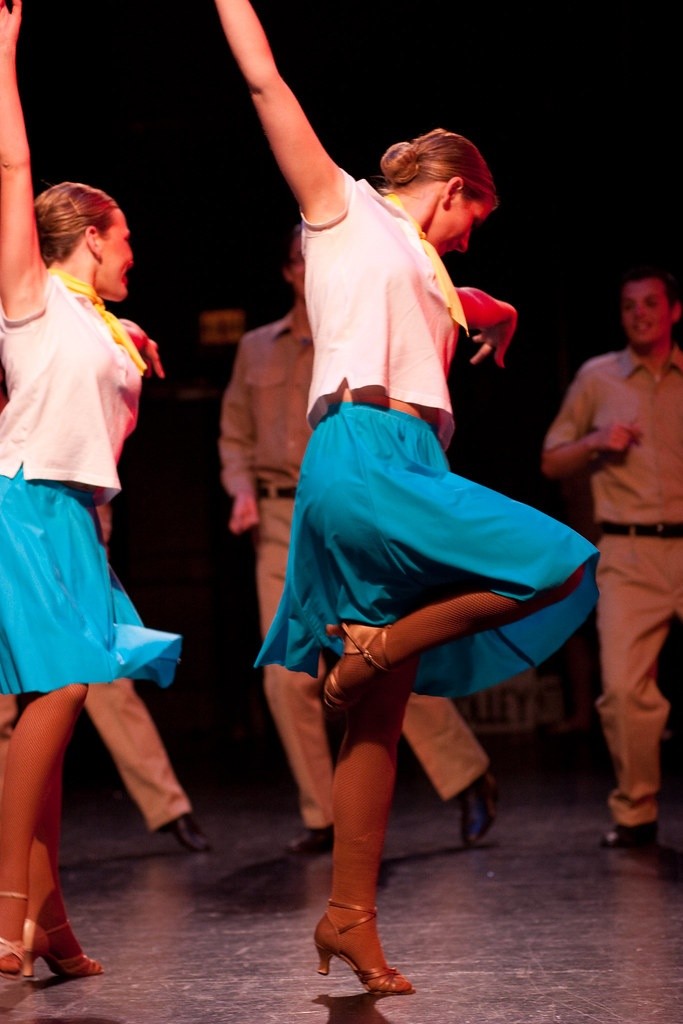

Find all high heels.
xmin=22 ymin=917 xmax=104 ymax=976
xmin=0 ymin=889 xmax=28 ymax=981
xmin=321 ymin=624 xmax=393 ymax=711
xmin=314 ymin=901 xmax=416 ymax=995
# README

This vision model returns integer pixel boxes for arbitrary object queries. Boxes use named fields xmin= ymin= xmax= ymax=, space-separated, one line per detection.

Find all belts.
xmin=599 ymin=521 xmax=683 ymax=538
xmin=256 ymin=486 xmax=298 ymax=500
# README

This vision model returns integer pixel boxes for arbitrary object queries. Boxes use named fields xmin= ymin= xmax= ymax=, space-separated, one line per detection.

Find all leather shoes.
xmin=161 ymin=815 xmax=206 ymax=849
xmin=601 ymin=820 xmax=658 ymax=847
xmin=457 ymin=771 xmax=508 ymax=839
xmin=301 ymin=825 xmax=340 ymax=855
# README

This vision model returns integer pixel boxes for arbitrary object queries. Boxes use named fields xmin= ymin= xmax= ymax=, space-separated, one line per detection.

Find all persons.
xmin=1 ymin=0 xmax=212 ymax=979
xmin=542 ymin=272 xmax=682 ymax=848
xmin=214 ymin=0 xmax=601 ymax=997
xmin=220 ymin=223 xmax=498 ymax=856
